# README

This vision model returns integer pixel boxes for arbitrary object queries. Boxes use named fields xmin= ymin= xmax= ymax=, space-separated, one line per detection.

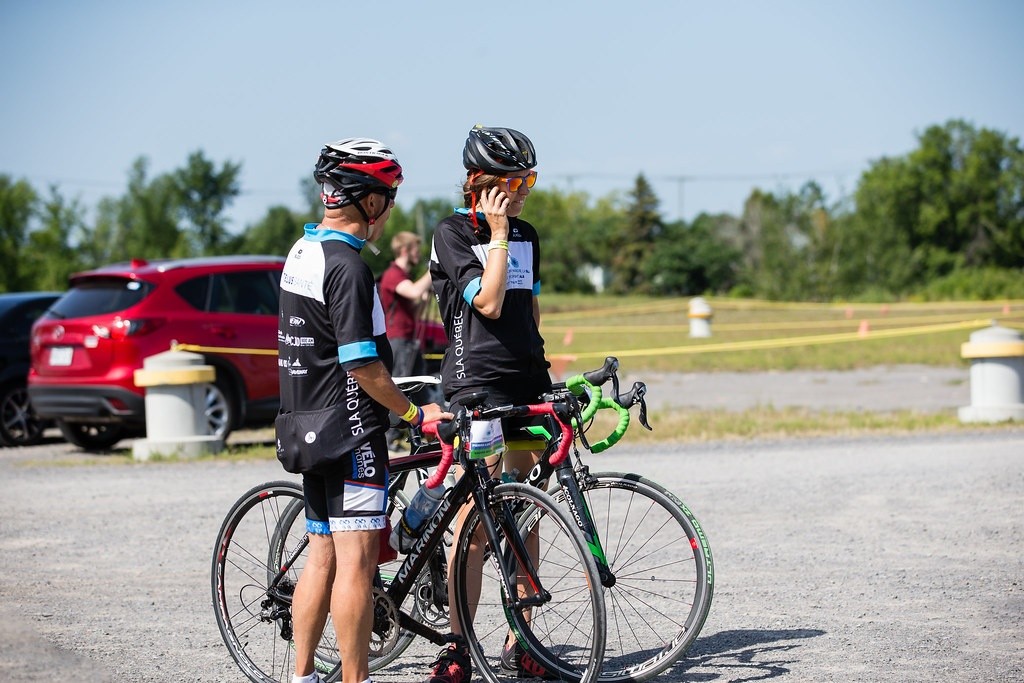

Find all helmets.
xmin=462 ymin=125 xmax=537 ymax=174
xmin=313 ymin=138 xmax=404 ymax=188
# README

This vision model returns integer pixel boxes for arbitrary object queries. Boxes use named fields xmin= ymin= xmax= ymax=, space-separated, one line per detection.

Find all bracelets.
xmin=487 ymin=240 xmax=509 ymax=251
xmin=398 ymin=400 xmax=418 ymax=422
xmin=408 ymin=404 xmax=424 ymax=430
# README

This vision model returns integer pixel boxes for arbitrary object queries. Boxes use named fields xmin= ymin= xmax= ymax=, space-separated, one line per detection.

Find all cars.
xmin=0 ymin=288 xmax=68 ymax=448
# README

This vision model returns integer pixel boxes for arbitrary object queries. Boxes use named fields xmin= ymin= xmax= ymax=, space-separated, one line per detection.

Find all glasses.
xmin=368 ymin=188 xmax=398 ymax=199
xmin=498 ymin=170 xmax=538 ymax=192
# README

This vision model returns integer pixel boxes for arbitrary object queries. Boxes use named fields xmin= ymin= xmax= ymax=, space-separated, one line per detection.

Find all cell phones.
xmin=485 ymin=186 xmax=509 ymax=210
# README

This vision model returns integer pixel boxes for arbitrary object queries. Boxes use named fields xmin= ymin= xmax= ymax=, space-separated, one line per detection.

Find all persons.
xmin=276 ymin=135 xmax=457 ymax=682
xmin=430 ymin=123 xmax=580 ymax=683
xmin=379 ymin=232 xmax=434 ymax=456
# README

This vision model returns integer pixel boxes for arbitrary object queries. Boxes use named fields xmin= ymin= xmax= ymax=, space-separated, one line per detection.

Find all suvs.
xmin=25 ymin=255 xmax=449 ymax=452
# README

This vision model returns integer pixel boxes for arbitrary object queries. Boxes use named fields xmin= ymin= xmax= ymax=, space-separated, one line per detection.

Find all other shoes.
xmin=388 ymin=446 xmax=411 ymax=458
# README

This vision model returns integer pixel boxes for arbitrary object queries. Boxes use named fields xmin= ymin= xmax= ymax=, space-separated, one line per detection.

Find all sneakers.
xmin=424 ymin=646 xmax=471 ymax=683
xmin=501 ymin=643 xmax=560 ymax=680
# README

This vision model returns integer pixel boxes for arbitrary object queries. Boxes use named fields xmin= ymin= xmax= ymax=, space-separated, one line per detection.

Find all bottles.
xmin=501 ymin=469 xmax=519 ymax=484
xmin=387 ymin=481 xmax=446 ymax=549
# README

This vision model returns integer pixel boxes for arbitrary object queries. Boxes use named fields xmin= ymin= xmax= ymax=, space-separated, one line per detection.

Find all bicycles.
xmin=209 ymin=383 xmax=607 ymax=683
xmin=267 ymin=356 xmax=715 ymax=683
